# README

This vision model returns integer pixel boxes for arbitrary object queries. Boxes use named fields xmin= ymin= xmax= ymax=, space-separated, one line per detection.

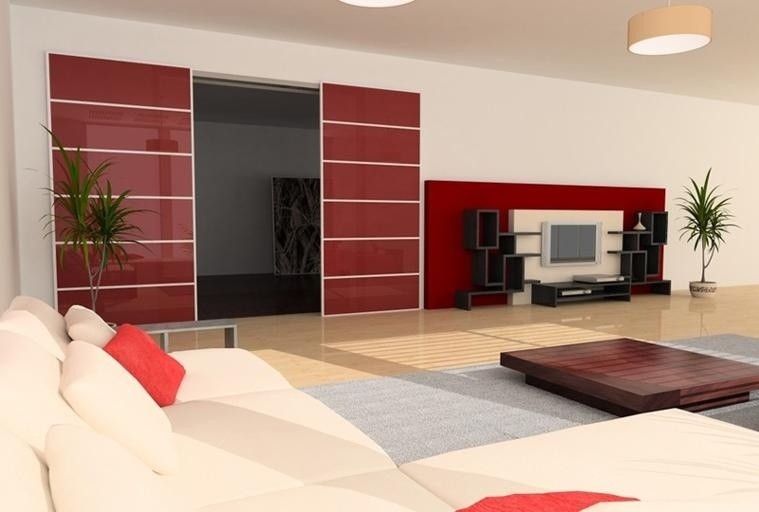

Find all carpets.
xmin=297 ymin=333 xmax=759 ymax=464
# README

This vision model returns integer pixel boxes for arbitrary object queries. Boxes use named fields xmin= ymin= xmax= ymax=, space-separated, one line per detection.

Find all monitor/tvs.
xmin=541 ymin=220 xmax=603 ymax=267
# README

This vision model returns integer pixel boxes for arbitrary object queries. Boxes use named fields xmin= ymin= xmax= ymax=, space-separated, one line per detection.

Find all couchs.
xmin=1 ymin=296 xmax=759 ymax=512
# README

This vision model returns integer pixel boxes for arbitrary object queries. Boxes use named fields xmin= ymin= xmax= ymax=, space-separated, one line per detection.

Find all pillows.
xmin=45 ymin=303 xmax=186 ymax=512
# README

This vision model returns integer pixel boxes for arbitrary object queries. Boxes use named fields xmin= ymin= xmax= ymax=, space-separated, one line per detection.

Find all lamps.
xmin=627 ymin=0 xmax=713 ymax=57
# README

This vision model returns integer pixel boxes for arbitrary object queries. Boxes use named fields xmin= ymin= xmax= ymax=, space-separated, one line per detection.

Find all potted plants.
xmin=674 ymin=167 xmax=743 ymax=298
xmin=30 ymin=122 xmax=161 ymax=329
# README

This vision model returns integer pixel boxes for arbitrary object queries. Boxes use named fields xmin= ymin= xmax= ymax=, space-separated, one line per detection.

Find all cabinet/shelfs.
xmin=456 ymin=209 xmax=672 ymax=311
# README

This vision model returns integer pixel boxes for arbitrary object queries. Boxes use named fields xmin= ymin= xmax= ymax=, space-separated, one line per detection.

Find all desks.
xmin=135 ymin=318 xmax=237 ymax=353
